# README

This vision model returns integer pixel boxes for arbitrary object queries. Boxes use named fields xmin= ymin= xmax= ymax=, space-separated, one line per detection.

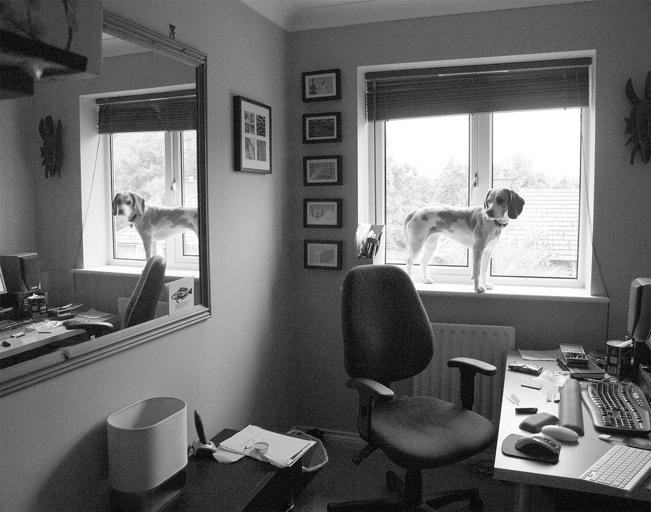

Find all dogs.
xmin=403 ymin=188 xmax=525 ymax=293
xmin=112 ymin=192 xmax=198 ymax=264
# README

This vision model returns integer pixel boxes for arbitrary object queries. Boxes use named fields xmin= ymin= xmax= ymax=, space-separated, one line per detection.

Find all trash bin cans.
xmin=284 ymin=429 xmax=328 ymax=497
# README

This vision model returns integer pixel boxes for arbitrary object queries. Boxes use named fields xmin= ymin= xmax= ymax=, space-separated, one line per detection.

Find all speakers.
xmin=626 ymin=277 xmax=651 ymax=342
xmin=4 ymin=253 xmax=40 ymax=289
xmin=12 ymin=292 xmax=25 ymax=321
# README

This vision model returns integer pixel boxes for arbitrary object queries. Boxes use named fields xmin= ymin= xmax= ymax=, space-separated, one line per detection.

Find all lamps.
xmin=0 ymin=0 xmax=104 ymax=83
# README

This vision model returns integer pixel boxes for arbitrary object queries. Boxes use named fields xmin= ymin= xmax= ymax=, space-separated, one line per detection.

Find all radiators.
xmin=117 ymin=296 xmax=168 ymax=330
xmin=390 ymin=322 xmax=516 ymax=431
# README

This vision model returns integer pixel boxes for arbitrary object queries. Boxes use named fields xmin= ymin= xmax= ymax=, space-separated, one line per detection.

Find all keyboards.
xmin=1 ymin=319 xmax=31 ymax=332
xmin=580 ymin=445 xmax=649 ymax=493
xmin=581 ymin=370 xmax=651 ymax=433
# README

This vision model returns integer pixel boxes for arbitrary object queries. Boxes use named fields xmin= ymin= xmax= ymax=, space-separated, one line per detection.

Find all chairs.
xmin=325 ymin=266 xmax=498 ymax=512
xmin=61 ymin=255 xmax=166 ymax=332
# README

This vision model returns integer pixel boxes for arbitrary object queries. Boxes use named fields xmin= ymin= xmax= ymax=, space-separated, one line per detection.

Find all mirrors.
xmin=1 ymin=8 xmax=212 ymax=396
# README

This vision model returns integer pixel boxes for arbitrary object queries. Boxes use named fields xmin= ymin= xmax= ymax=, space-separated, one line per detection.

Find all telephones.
xmin=193 ymin=410 xmax=216 ymax=458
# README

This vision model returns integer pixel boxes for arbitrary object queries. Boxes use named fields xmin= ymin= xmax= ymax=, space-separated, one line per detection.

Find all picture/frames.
xmin=302 ymin=112 xmax=342 ymax=144
xmin=303 ymin=198 xmax=342 ymax=229
xmin=303 ymin=240 xmax=342 ymax=271
xmin=234 ymin=95 xmax=272 ymax=174
xmin=303 ymin=155 xmax=343 ymax=186
xmin=302 ymin=69 xmax=341 ymax=103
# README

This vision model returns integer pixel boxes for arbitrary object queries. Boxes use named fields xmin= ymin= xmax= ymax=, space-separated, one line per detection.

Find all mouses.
xmin=514 ymin=431 xmax=565 ymax=458
xmin=542 ymin=422 xmax=577 ymax=443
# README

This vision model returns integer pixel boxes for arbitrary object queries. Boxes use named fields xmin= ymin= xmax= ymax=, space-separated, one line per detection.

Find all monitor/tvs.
xmin=1 ymin=268 xmax=7 ymax=296
xmin=645 ymin=330 xmax=651 ymax=349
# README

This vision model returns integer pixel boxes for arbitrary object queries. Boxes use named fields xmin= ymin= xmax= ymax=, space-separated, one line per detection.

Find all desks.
xmin=493 ymin=351 xmax=650 ymax=511
xmin=52 ymin=427 xmax=302 ymax=512
xmin=0 ymin=312 xmax=87 ymax=362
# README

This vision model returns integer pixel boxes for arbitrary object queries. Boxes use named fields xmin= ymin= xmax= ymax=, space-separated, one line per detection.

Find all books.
xmin=556 ymin=357 xmax=606 ymax=380
xmin=218 ymin=424 xmax=317 ymax=469
